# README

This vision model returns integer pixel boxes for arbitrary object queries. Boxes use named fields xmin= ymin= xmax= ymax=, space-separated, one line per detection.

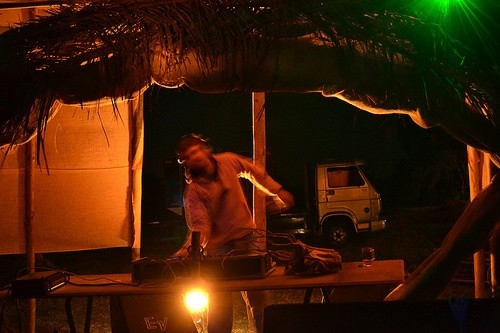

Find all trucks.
xmin=141 ymin=156 xmax=389 ymax=265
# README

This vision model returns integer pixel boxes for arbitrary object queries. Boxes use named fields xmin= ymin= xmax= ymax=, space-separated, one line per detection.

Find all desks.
xmin=0 ymin=259 xmax=405 ymax=333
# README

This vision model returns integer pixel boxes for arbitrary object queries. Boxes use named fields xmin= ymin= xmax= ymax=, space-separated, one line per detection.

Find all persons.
xmin=171 ymin=132 xmax=295 ymax=260
